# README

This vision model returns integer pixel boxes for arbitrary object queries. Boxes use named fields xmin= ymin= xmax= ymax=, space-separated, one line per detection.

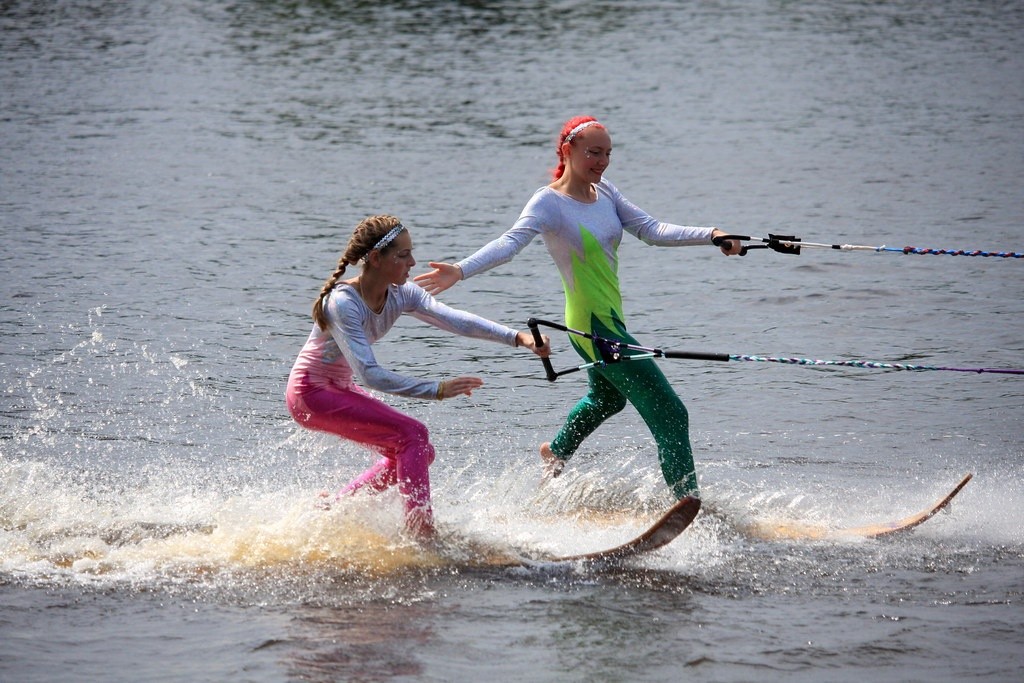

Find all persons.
xmin=412 ymin=115 xmax=741 ymax=504
xmin=286 ymin=214 xmax=551 ymax=542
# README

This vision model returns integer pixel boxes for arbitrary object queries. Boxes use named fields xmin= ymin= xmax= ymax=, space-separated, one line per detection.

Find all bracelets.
xmin=437 ymin=381 xmax=445 ymax=401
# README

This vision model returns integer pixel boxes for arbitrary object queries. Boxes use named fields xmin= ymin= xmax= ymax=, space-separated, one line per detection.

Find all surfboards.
xmin=753 ymin=472 xmax=972 ymax=540
xmin=484 ymin=496 xmax=700 ymax=567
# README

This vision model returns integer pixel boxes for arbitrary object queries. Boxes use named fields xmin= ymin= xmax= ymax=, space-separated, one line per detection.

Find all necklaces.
xmin=358 ymin=276 xmax=385 ymax=313
